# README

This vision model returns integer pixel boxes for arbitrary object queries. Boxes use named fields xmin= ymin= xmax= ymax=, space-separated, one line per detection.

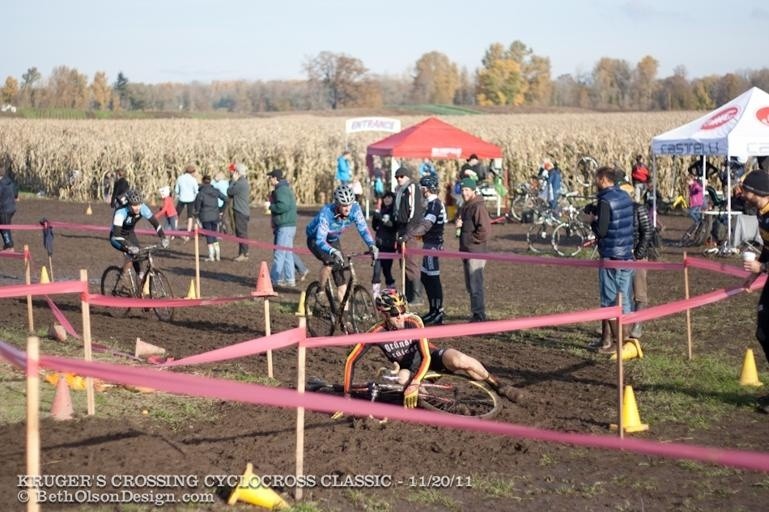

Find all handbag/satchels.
xmin=632 ymin=167 xmax=648 ymax=182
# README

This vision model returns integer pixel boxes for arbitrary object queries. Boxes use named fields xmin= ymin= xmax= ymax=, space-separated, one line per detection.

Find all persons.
xmin=576 ymin=153 xmax=767 ymax=414
xmin=292 ymin=252 xmax=310 ymax=282
xmin=153 ymin=163 xmax=251 ymax=263
xmin=306 ymin=184 xmax=380 ymax=314
xmin=330 ymin=287 xmax=525 ymax=419
xmin=336 ymin=150 xmax=560 ymax=326
xmin=0 ymin=167 xmax=18 ymax=250
xmin=109 ymin=191 xmax=170 ymax=313
xmin=111 ymin=169 xmax=129 ymax=208
xmin=266 ymin=169 xmax=308 ymax=290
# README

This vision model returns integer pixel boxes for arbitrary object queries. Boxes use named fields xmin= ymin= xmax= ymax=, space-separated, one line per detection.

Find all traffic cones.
xmin=134 ymin=336 xmax=166 ymax=359
xmin=149 ymin=194 xmax=157 ymax=207
xmin=46 ymin=372 xmax=80 ymax=424
xmin=47 ymin=320 xmax=68 ymax=344
xmin=227 ymin=462 xmax=295 ymax=511
xmin=295 ymin=289 xmax=313 ymax=317
xmin=609 ymin=385 xmax=648 ymax=432
xmin=183 ymin=279 xmax=197 ymax=300
xmin=250 ymin=259 xmax=279 ymax=297
xmin=734 ymin=347 xmax=763 ymax=386
xmin=85 ymin=202 xmax=92 ymax=216
xmin=39 ymin=266 xmax=51 ymax=283
xmin=610 ymin=338 xmax=643 ymax=361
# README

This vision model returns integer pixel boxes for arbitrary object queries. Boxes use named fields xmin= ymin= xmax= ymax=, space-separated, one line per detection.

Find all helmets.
xmin=376 ymin=288 xmax=408 ymax=312
xmin=333 ymin=184 xmax=355 ymax=206
xmin=126 ymin=190 xmax=142 ymax=205
xmin=420 ymin=177 xmax=438 ymax=188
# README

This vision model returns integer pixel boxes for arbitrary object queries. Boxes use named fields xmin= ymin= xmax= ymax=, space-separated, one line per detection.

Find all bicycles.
xmin=305 ymin=361 xmax=503 ymax=425
xmin=101 ymin=241 xmax=175 ymax=322
xmin=304 ymin=247 xmax=379 ymax=337
xmin=509 ymin=153 xmax=599 ymax=261
xmin=701 ymin=240 xmax=740 ymax=259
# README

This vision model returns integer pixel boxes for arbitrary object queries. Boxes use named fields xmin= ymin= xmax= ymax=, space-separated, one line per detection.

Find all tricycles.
xmin=656 ymin=190 xmax=683 ymax=215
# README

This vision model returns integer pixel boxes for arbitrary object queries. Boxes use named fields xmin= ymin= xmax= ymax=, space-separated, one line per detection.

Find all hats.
xmin=266 ymin=169 xmax=282 ymax=177
xmin=461 ymin=178 xmax=475 ymax=190
xmin=742 ymin=170 xmax=769 ymax=196
xmin=395 ymin=168 xmax=412 ymax=179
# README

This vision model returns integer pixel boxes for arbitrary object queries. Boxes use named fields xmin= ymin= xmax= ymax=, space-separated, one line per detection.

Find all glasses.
xmin=383 ymin=305 xmax=407 ymax=316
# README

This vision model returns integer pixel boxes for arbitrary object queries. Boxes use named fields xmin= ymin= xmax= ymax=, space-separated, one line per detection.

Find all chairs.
xmin=730 ymin=214 xmax=761 ymax=254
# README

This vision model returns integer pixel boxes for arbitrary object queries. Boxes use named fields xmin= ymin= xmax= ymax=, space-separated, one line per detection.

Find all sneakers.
xmin=117 ymin=271 xmax=131 ymax=290
xmin=408 ymin=299 xmax=424 ymax=306
xmin=314 ymin=289 xmax=330 ymax=308
xmin=301 ymin=269 xmax=309 ymax=282
xmin=233 ymin=253 xmax=248 ymax=262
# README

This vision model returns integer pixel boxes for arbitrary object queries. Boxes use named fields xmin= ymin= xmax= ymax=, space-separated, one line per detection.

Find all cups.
xmin=741 ymin=251 xmax=757 ymax=263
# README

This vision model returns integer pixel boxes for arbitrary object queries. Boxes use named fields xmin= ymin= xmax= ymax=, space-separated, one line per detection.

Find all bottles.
xmin=583 ymin=239 xmax=596 ymax=249
xmin=455 ymin=212 xmax=461 ymax=239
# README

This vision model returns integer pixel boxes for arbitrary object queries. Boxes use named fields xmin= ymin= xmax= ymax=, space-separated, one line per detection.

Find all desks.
xmin=695 ymin=209 xmax=742 ymax=245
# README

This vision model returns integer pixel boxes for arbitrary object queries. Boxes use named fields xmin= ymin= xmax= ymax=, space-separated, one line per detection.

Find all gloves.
xmin=331 ymin=249 xmax=344 ymax=267
xmin=128 ymin=246 xmax=139 ymax=254
xmin=161 ymin=239 xmax=169 ymax=247
xmin=331 ymin=410 xmax=343 ymax=420
xmin=370 ymin=244 xmax=379 ymax=260
xmin=397 ymin=234 xmax=409 ymax=249
xmin=403 ymin=384 xmax=418 ymax=408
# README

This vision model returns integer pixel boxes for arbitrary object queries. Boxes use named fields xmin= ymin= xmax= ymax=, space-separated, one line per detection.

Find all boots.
xmin=586 ymin=301 xmax=647 ymax=354
xmin=205 ymin=242 xmax=221 ymax=262
xmin=486 ymin=373 xmax=526 ymax=403
xmin=422 ymin=298 xmax=443 ymax=325
